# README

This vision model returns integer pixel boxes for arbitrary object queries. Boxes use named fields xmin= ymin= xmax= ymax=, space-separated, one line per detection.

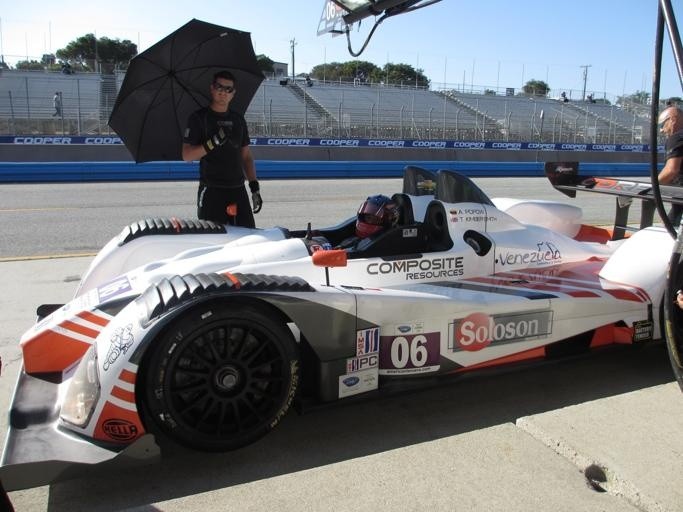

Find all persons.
xmin=306 ymin=76 xmax=313 ymax=87
xmin=561 ymin=92 xmax=569 ymax=103
xmin=585 ymin=95 xmax=596 ymax=104
xmin=380 ymin=81 xmax=384 ymax=88
xmin=484 ymin=89 xmax=496 ymax=96
xmin=447 ymin=90 xmax=453 ymax=97
xmin=181 ymin=73 xmax=263 ymax=228
xmin=333 ymin=193 xmax=410 ymax=259
xmin=636 ymin=106 xmax=683 ymax=226
xmin=507 ymin=90 xmax=513 ymax=96
xmin=50 ymin=92 xmax=62 ymax=120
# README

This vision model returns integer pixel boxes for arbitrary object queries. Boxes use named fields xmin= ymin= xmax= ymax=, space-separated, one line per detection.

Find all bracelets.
xmin=248 ymin=180 xmax=259 ymax=194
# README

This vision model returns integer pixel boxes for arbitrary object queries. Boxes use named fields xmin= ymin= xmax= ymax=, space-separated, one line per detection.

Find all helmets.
xmin=355 ymin=195 xmax=399 ymax=238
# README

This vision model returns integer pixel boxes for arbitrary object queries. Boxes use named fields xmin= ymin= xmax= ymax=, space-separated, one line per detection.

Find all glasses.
xmin=214 ymin=82 xmax=233 ymax=93
xmin=659 ymin=117 xmax=671 ymax=128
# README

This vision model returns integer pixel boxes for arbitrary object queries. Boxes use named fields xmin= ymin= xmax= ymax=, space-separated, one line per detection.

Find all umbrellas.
xmin=105 ymin=18 xmax=268 ymax=164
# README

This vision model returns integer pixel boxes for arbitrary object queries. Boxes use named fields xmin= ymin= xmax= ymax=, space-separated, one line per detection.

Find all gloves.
xmin=203 ymin=127 xmax=231 ymax=153
xmin=249 ymin=180 xmax=263 ymax=213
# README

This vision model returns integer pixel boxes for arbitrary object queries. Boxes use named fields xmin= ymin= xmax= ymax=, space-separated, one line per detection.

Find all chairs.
xmin=295 ymin=194 xmax=454 ymax=260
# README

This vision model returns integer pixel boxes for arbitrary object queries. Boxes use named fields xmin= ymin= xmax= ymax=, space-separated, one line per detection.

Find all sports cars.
xmin=19 ymin=161 xmax=683 ymax=453
